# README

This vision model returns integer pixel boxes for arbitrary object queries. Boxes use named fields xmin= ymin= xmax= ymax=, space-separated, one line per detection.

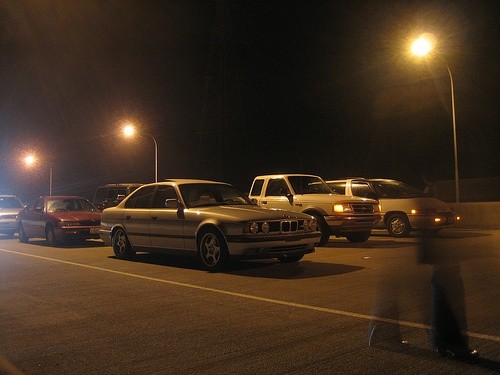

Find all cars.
xmin=16 ymin=196 xmax=102 ymax=247
xmin=100 ymin=179 xmax=322 ymax=273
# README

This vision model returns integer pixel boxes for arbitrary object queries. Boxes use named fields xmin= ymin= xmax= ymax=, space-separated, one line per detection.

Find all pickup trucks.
xmin=246 ymin=174 xmax=382 ymax=247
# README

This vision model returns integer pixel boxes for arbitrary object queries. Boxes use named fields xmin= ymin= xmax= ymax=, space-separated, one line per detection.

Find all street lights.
xmin=24 ymin=154 xmax=53 ymax=196
xmin=412 ymin=32 xmax=460 ymax=203
xmin=121 ymin=120 xmax=157 ymax=183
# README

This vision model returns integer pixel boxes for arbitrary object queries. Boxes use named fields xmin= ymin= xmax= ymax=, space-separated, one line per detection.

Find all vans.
xmin=308 ymin=177 xmax=462 ymax=238
xmin=92 ymin=183 xmax=145 ymax=210
xmin=0 ymin=194 xmax=28 ymax=238
